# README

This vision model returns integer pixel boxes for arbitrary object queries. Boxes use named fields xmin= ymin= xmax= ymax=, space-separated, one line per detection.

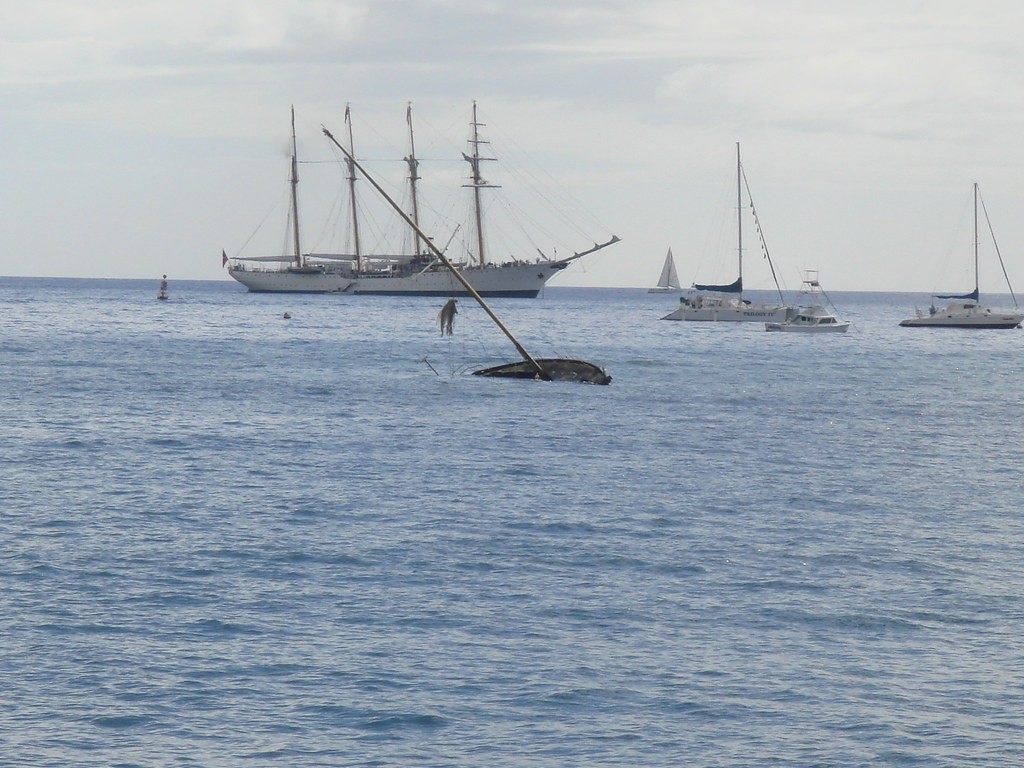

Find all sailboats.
xmin=896 ymin=182 xmax=1024 ymax=330
xmin=647 ymin=246 xmax=684 ymax=294
xmin=217 ymin=100 xmax=625 ymax=298
xmin=317 ymin=120 xmax=613 ymax=386
xmin=658 ymin=141 xmax=801 ymax=323
xmin=157 ymin=275 xmax=170 ymax=302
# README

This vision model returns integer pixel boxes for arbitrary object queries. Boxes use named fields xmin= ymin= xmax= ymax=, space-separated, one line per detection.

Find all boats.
xmin=763 ymin=269 xmax=850 ymax=333
